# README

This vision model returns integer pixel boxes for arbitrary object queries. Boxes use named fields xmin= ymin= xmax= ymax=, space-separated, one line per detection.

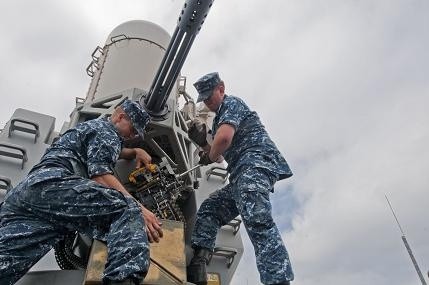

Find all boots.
xmin=187 ymin=247 xmax=212 ymax=285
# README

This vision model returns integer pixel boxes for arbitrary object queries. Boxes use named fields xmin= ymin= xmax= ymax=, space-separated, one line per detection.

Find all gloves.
xmin=199 ymin=152 xmax=214 ymax=165
xmin=188 ymin=123 xmax=208 ymax=147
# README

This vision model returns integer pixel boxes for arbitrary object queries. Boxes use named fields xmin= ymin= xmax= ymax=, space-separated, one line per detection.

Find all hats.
xmin=120 ymin=99 xmax=151 ymax=137
xmin=193 ymin=72 xmax=221 ymax=104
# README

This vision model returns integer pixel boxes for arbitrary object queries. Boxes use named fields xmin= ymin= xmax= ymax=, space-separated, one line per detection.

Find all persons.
xmin=187 ymin=73 xmax=295 ymax=285
xmin=0 ymin=99 xmax=164 ymax=285
